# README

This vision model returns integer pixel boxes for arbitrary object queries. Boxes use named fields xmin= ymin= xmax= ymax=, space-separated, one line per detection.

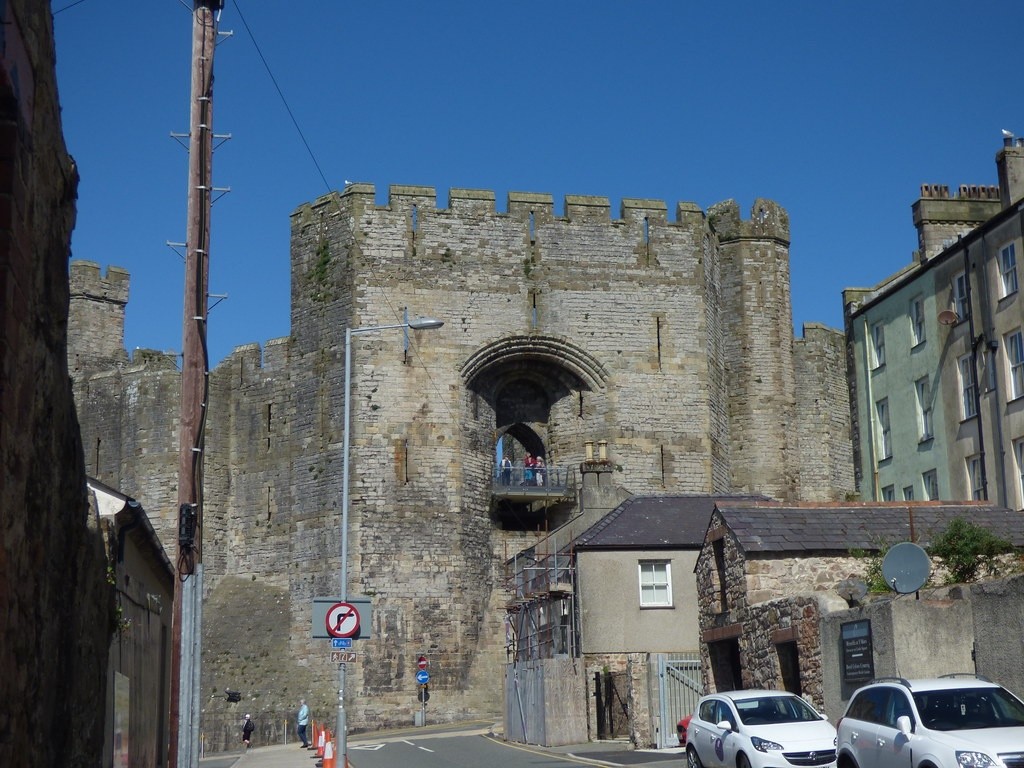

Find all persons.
xmin=242 ymin=714 xmax=252 ymax=748
xmin=676 ymin=716 xmax=692 ymax=746
xmin=525 ymin=453 xmax=544 ymax=486
xmin=297 ymin=700 xmax=309 ymax=748
xmin=499 ymin=453 xmax=513 ymax=486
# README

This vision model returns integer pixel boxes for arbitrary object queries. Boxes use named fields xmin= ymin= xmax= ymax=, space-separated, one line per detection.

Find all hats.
xmin=504 ymin=453 xmax=509 ymax=457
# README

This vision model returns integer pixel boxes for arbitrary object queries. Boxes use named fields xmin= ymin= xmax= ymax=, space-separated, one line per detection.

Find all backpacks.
xmin=247 ymin=721 xmax=254 ymax=732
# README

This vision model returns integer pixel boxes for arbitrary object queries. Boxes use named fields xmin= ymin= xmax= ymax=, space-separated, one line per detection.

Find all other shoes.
xmin=300 ymin=745 xmax=307 ymax=748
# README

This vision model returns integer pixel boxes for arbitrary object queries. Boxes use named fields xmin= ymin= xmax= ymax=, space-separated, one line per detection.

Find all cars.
xmin=676 ymin=711 xmax=695 ymax=747
xmin=684 ymin=689 xmax=841 ymax=768
xmin=836 ymin=673 xmax=1024 ymax=768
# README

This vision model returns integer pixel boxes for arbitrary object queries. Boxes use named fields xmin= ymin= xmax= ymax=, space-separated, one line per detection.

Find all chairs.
xmin=751 ymin=705 xmax=777 ymax=721
xmin=959 ymin=696 xmax=990 ymax=726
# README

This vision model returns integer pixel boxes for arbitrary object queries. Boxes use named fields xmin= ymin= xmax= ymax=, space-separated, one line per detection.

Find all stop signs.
xmin=418 ymin=656 xmax=427 ymax=668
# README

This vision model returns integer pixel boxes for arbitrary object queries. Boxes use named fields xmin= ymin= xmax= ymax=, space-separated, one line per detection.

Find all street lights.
xmin=331 ymin=318 xmax=446 ymax=768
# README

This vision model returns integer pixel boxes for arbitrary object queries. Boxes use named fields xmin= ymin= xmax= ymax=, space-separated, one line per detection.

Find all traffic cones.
xmin=320 ymin=727 xmax=333 ymax=768
xmin=306 ymin=722 xmax=325 ymax=758
xmin=331 ymin=737 xmax=338 ymax=768
